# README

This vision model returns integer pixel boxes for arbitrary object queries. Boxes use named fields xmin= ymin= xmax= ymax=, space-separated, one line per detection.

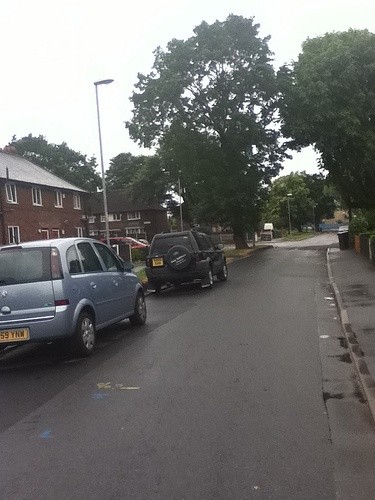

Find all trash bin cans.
xmin=320 ymin=228 xmax=322 ymax=231
xmin=337 ymin=231 xmax=349 ymax=249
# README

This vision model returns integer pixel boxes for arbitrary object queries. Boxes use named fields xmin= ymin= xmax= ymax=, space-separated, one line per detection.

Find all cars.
xmin=0 ymin=238 xmax=146 ymax=358
xmin=338 ymin=226 xmax=349 ymax=234
xmin=99 ymin=237 xmax=149 ymax=259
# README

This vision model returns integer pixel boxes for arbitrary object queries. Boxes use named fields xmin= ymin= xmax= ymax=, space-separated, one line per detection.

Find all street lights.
xmin=287 ymin=194 xmax=292 ymax=239
xmin=93 ymin=80 xmax=115 ymax=245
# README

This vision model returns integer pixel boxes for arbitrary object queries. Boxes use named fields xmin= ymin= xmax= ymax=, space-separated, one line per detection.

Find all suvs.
xmin=144 ymin=231 xmax=228 ymax=290
